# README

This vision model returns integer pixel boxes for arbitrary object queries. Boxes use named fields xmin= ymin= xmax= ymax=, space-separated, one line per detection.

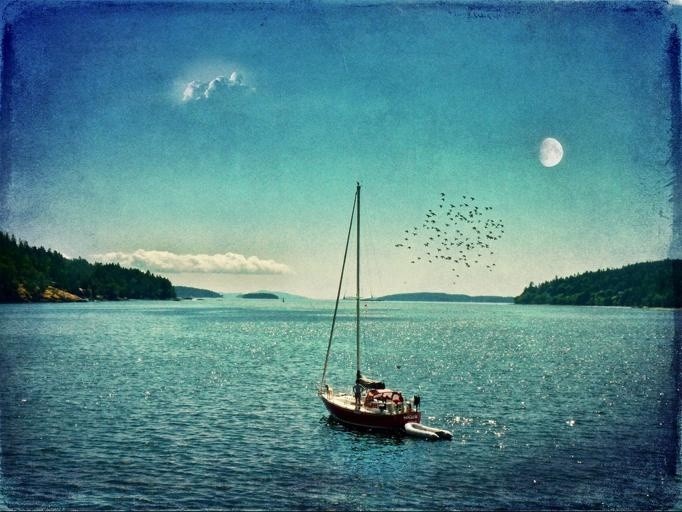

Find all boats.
xmin=405 ymin=421 xmax=454 ymax=441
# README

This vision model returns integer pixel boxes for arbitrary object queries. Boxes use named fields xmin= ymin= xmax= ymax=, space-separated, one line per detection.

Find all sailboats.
xmin=315 ymin=180 xmax=422 ymax=432
